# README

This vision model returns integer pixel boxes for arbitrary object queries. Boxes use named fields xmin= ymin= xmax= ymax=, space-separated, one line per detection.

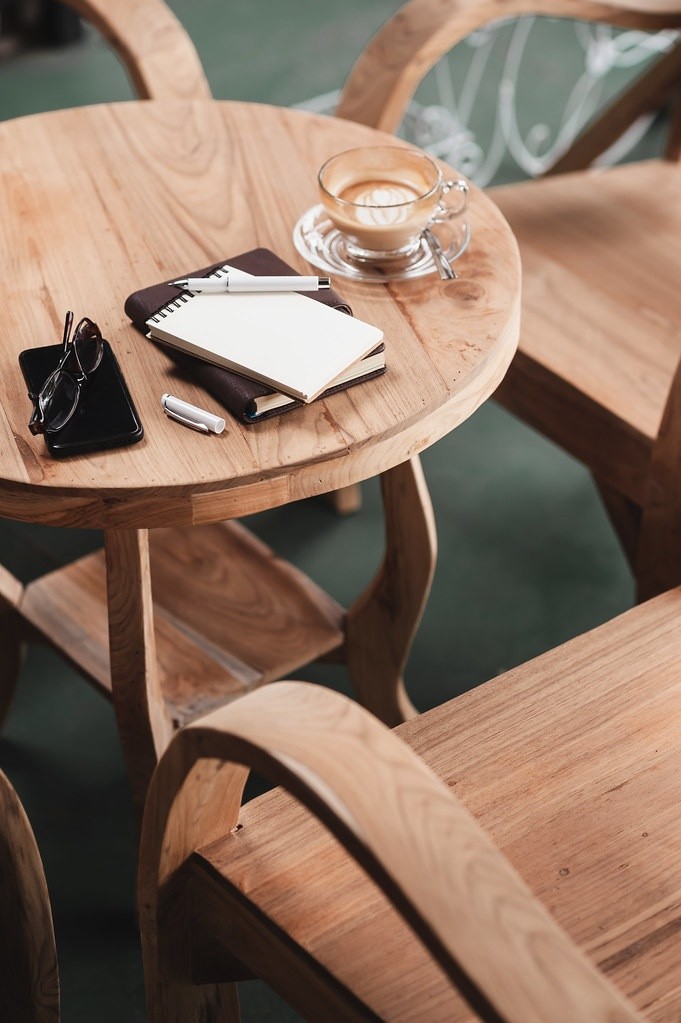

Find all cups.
xmin=318 ymin=146 xmax=473 ymax=263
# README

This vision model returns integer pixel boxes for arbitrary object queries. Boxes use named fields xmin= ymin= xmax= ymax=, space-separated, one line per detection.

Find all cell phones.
xmin=20 ymin=339 xmax=144 ymax=456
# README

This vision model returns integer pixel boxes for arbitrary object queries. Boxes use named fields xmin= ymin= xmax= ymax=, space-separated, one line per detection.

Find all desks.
xmin=1 ymin=100 xmax=524 ymax=823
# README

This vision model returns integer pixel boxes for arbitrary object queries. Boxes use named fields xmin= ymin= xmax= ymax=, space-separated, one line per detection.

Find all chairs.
xmin=318 ymin=1 xmax=681 ymax=608
xmin=134 ymin=588 xmax=681 ymax=1023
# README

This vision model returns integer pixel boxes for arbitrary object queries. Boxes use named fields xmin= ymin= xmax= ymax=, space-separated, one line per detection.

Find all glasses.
xmin=27 ymin=310 xmax=106 ymax=434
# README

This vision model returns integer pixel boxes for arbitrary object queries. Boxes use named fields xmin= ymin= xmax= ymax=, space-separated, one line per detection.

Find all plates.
xmin=293 ymin=203 xmax=470 ymax=283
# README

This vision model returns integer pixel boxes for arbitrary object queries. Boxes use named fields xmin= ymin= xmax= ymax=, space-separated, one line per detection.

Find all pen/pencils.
xmin=167 ymin=275 xmax=331 ymax=293
